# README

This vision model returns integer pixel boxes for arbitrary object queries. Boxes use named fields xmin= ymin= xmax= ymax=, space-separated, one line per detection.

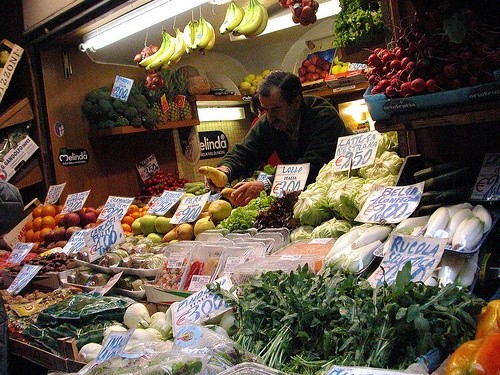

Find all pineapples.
xmin=147 ymin=68 xmax=192 ymax=124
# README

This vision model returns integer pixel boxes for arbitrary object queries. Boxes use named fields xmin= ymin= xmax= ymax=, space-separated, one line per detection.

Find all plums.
xmin=297 ymin=55 xmax=331 ymax=82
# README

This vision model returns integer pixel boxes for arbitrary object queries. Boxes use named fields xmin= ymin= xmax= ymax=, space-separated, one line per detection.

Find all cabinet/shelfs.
xmin=0 ymin=0 xmax=500 ymax=374
xmin=0 ymin=0 xmax=500 ymax=179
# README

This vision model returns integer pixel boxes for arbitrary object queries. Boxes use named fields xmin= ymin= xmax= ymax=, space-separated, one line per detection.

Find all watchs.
xmin=263 ymin=180 xmax=271 ymax=195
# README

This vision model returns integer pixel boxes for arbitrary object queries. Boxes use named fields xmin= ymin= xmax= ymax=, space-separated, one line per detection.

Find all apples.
xmin=238 ymin=69 xmax=273 ymax=96
xmin=37 ymin=207 xmax=105 ymax=249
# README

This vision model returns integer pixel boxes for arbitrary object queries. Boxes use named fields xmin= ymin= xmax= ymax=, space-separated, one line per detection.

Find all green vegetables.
xmin=206 ymin=262 xmax=488 ymax=375
xmin=22 ymin=295 xmax=127 ymax=350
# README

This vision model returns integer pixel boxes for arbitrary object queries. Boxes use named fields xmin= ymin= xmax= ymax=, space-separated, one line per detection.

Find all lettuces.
xmin=216 ymin=177 xmax=274 ymax=232
xmin=331 ymin=0 xmax=385 ymax=53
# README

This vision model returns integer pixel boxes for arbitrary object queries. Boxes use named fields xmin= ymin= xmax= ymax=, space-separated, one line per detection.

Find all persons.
xmin=204 ymin=70 xmax=349 ymax=207
xmin=0 ymin=178 xmax=24 ymax=375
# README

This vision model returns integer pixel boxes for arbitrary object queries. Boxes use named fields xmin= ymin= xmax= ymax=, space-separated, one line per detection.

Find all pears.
xmin=132 ymin=194 xmax=232 ymax=242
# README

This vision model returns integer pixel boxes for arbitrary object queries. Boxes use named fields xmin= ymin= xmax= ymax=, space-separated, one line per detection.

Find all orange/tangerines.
xmin=23 ymin=203 xmax=65 ymax=251
xmin=120 ymin=204 xmax=149 ymax=235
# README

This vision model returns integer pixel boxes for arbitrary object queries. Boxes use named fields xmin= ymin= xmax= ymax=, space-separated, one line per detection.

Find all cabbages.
xmin=324 ymin=203 xmax=492 ymax=287
xmin=289 ymin=133 xmax=403 ymax=242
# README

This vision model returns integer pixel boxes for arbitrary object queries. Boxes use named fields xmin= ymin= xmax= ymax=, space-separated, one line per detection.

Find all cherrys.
xmin=134 ymin=168 xmax=191 ymax=204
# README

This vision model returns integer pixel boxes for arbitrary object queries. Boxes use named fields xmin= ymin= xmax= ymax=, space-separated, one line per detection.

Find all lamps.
xmin=228 ymin=1 xmax=342 ymax=42
xmin=77 ymin=0 xmax=224 ymax=53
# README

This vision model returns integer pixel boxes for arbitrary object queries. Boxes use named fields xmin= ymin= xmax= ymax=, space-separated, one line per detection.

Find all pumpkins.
xmin=175 ymin=66 xmax=210 ymax=95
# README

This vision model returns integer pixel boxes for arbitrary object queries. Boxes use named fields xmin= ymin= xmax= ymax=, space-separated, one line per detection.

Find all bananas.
xmin=219 ymin=0 xmax=268 ymax=39
xmin=138 ymin=16 xmax=215 ymax=70
